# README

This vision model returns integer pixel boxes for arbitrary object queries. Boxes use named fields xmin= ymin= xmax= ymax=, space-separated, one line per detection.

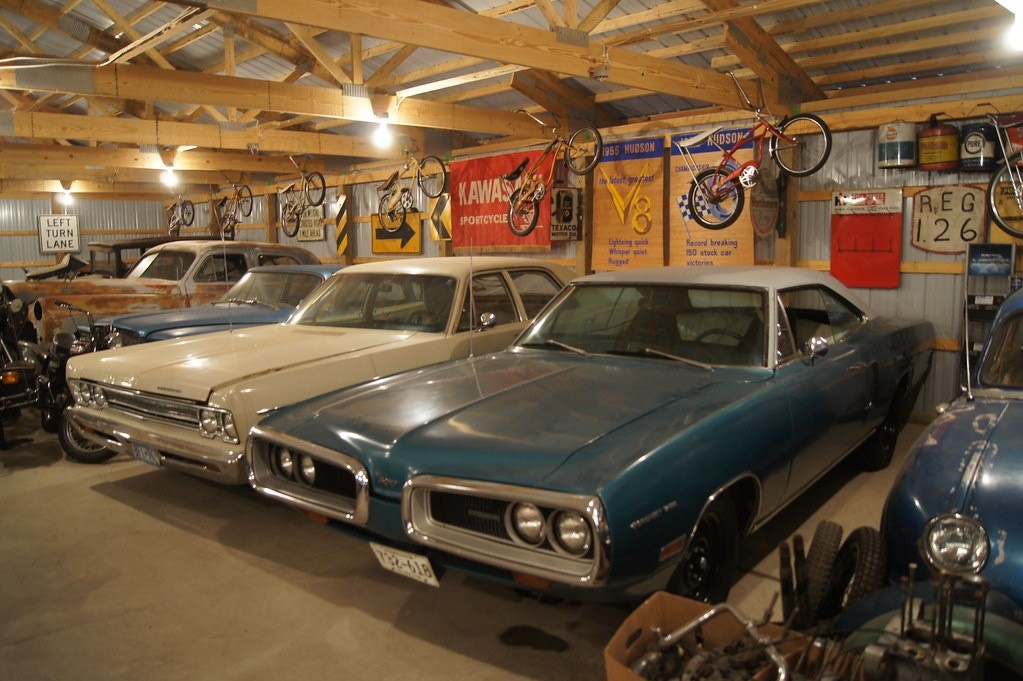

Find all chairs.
xmin=674 ymin=308 xmax=860 ymax=357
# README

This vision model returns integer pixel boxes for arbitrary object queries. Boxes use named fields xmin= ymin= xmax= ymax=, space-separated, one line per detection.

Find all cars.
xmin=2 ymin=236 xmax=326 ymax=322
xmin=93 ymin=263 xmax=346 ymax=355
xmin=64 ymin=252 xmax=581 ymax=497
xmin=878 ymin=286 xmax=1023 ymax=675
xmin=244 ymin=263 xmax=939 ymax=613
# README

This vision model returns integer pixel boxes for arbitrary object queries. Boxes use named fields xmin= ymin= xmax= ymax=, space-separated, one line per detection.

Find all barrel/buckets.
xmin=960 ymin=124 xmax=998 ymax=172
xmin=878 ymin=119 xmax=918 ymax=167
xmin=1006 ymin=126 xmax=1023 ymax=170
xmin=918 ymin=112 xmax=960 ymax=170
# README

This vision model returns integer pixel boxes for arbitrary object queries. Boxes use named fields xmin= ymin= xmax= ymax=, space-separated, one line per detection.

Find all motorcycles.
xmin=0 ymin=275 xmax=131 ymax=463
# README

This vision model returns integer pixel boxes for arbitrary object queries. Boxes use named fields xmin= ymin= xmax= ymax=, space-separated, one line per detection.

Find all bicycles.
xmin=376 ymin=133 xmax=447 ymax=233
xmin=278 ymin=152 xmax=326 ymax=238
xmin=677 ymin=66 xmax=833 ymax=230
xmin=166 ymin=184 xmax=196 ymax=238
xmin=987 ymin=111 xmax=1023 ymax=240
xmin=215 ymin=170 xmax=254 ymax=242
xmin=498 ymin=108 xmax=605 ymax=237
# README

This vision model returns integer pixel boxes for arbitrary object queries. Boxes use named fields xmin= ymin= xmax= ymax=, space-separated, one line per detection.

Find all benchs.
xmin=465 ymin=301 xmax=546 ymax=327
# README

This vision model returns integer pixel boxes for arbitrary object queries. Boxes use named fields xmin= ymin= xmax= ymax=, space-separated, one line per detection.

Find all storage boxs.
xmin=604 ymin=591 xmax=860 ymax=681
